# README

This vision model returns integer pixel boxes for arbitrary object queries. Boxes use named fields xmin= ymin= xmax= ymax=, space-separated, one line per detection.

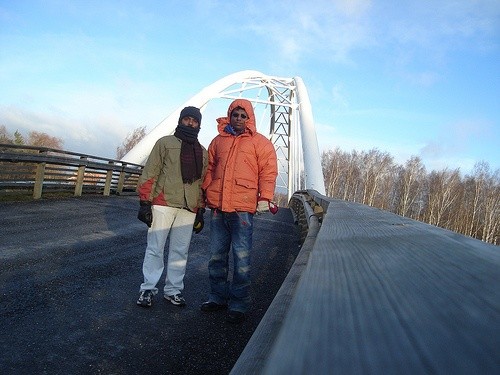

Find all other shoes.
xmin=201 ymin=301 xmax=214 ymax=312
xmin=224 ymin=311 xmax=245 ymax=323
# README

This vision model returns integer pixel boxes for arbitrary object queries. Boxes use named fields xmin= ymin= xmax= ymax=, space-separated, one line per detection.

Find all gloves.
xmin=255 ymin=200 xmax=270 ymax=215
xmin=138 ymin=200 xmax=152 ymax=228
xmin=193 ymin=208 xmax=205 ymax=234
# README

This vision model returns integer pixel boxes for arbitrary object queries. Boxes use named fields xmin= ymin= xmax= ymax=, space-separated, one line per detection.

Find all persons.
xmin=136 ymin=106 xmax=208 ymax=307
xmin=201 ymin=99 xmax=278 ymax=324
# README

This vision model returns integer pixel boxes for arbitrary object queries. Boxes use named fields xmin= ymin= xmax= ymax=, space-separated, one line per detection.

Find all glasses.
xmin=231 ymin=113 xmax=248 ymax=118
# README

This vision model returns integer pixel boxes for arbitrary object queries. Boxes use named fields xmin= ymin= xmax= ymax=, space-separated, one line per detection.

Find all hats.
xmin=178 ymin=106 xmax=201 ymax=128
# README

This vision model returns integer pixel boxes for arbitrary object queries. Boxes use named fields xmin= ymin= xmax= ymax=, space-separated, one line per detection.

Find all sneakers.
xmin=163 ymin=294 xmax=185 ymax=305
xmin=137 ymin=290 xmax=152 ymax=306
